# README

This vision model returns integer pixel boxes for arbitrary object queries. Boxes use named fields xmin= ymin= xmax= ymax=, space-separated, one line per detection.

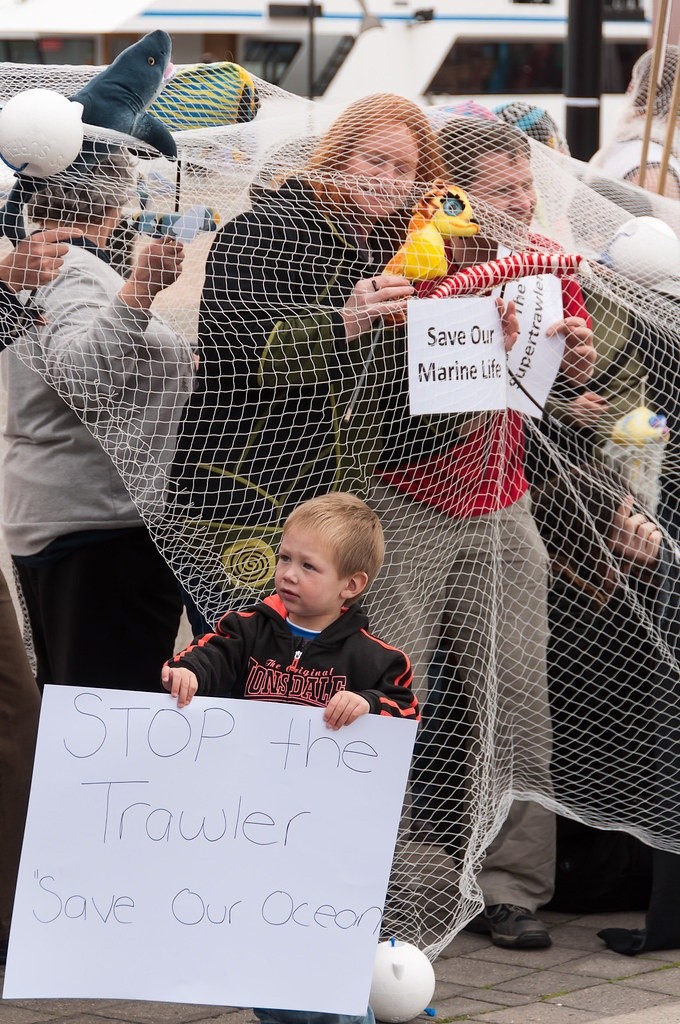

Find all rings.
xmin=371 ymin=280 xmax=378 ymax=291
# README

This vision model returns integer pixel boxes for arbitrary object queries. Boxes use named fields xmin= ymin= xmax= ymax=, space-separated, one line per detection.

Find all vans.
xmin=281 ymin=8 xmax=660 ymax=167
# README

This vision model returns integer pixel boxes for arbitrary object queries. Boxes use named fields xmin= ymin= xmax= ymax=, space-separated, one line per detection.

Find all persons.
xmin=0 ymin=46 xmax=680 ymax=1022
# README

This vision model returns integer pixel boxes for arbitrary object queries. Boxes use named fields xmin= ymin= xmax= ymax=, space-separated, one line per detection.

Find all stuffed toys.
xmin=385 ymin=176 xmax=582 ymax=329
xmin=0 ymin=30 xmax=176 ymax=242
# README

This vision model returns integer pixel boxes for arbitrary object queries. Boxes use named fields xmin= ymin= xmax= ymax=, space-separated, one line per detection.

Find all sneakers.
xmin=464 ymin=904 xmax=552 ymax=949
